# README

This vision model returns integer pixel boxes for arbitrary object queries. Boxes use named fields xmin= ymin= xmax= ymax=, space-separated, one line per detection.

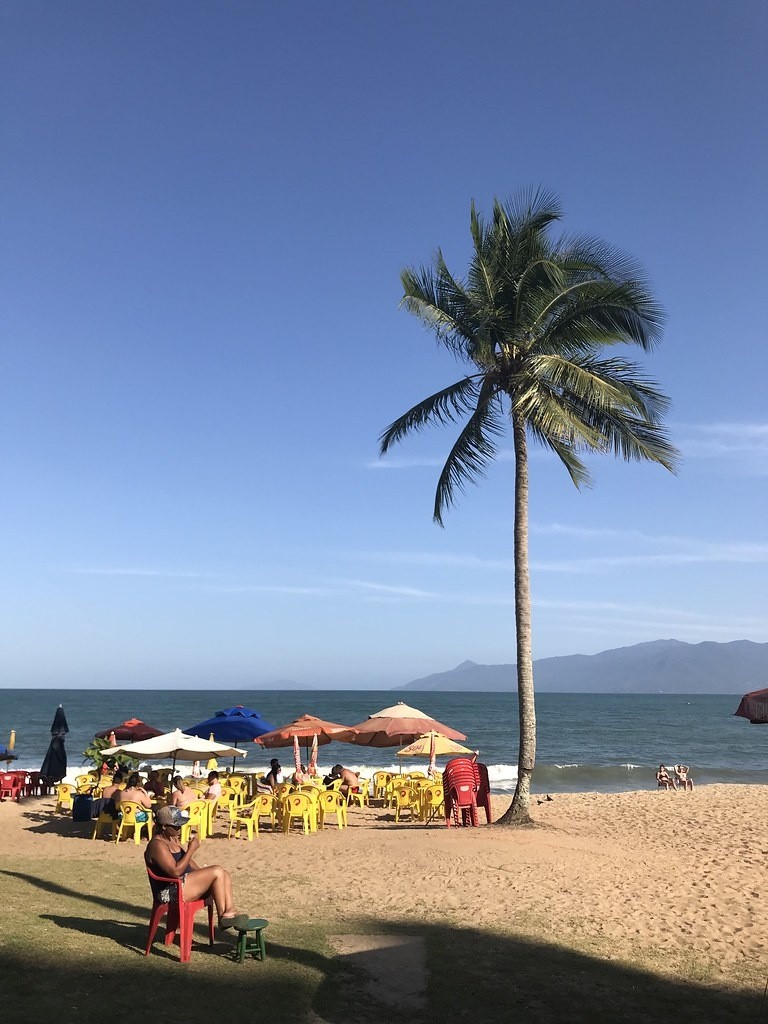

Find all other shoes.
xmin=221 ymin=913 xmax=248 ymax=927
xmin=218 ymin=922 xmax=232 ymax=931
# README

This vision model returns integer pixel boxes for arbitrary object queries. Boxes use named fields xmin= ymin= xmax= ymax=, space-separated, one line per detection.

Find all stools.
xmin=233 ymin=917 xmax=269 ymax=965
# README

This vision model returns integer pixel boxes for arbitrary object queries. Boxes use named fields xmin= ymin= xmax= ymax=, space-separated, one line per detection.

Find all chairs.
xmin=0 ymin=771 xmax=56 ymax=803
xmin=372 ymin=758 xmax=493 ymax=829
xmin=75 ymin=769 xmax=371 ymax=841
xmin=54 ymin=784 xmax=78 ymax=816
xmin=318 ymin=791 xmax=348 ymax=830
xmin=655 ymin=769 xmax=673 ymax=791
xmin=674 ymin=765 xmax=690 ymax=790
xmin=143 ymin=866 xmax=215 ymax=963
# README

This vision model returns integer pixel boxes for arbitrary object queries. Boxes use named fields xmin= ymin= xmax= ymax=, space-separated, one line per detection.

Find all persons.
xmin=145 ymin=806 xmax=250 ymax=932
xmin=260 ymin=758 xmax=359 ymax=806
xmin=103 ymin=757 xmax=222 ymax=840
xmin=656 ymin=763 xmax=694 ymax=791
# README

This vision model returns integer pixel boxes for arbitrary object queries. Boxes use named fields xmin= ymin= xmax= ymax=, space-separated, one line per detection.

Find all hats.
xmin=157 ymin=805 xmax=190 ymax=826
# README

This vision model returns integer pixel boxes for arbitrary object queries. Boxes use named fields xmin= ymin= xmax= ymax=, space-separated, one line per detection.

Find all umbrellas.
xmin=95 ymin=702 xmax=480 ymax=794
xmin=42 ymin=702 xmax=70 ymax=793
xmin=730 ymin=688 xmax=768 ymax=724
xmin=0 ymin=729 xmax=19 ymax=772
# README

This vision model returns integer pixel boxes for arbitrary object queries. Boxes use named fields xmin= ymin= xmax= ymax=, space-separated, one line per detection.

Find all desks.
xmin=232 ymin=770 xmax=258 ymax=800
